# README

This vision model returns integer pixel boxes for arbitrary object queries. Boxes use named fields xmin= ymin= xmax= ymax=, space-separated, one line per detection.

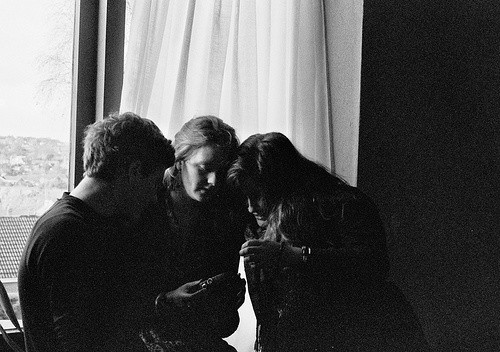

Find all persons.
xmin=228 ymin=132 xmax=436 ymax=351
xmin=16 ymin=110 xmax=176 ymax=351
xmin=118 ymin=117 xmax=249 ymax=351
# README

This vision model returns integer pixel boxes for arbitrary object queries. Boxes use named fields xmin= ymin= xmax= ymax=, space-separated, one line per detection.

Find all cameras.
xmin=198 ymin=274 xmax=245 ymax=338
